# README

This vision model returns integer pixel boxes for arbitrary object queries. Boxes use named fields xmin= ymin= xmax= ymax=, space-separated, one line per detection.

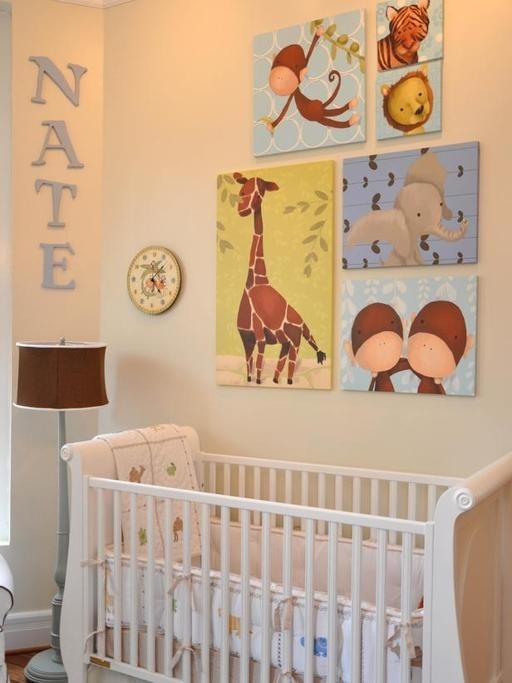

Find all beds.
xmin=59 ymin=425 xmax=512 ymax=683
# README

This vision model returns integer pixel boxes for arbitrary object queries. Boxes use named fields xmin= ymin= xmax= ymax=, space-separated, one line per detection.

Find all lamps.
xmin=13 ymin=336 xmax=109 ymax=683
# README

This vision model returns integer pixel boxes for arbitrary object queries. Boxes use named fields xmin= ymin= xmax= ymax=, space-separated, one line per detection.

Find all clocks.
xmin=127 ymin=245 xmax=181 ymax=315
